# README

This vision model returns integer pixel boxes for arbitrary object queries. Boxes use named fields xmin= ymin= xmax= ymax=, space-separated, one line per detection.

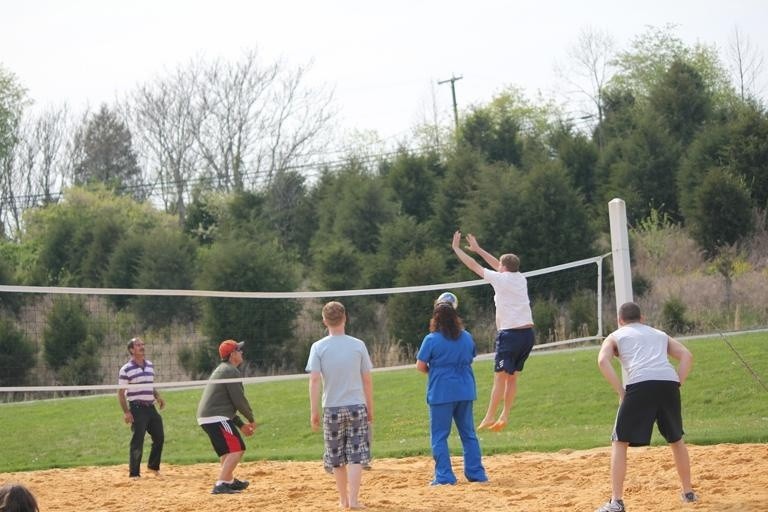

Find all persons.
xmin=450 ymin=229 xmax=536 ymax=433
xmin=0 ymin=483 xmax=40 ymax=512
xmin=115 ymin=337 xmax=167 ymax=480
xmin=193 ymin=338 xmax=258 ymax=495
xmin=592 ymin=300 xmax=700 ymax=510
xmin=303 ymin=300 xmax=376 ymax=510
xmin=416 ymin=298 xmax=490 ymax=486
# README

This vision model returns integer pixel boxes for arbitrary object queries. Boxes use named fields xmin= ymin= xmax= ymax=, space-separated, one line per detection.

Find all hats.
xmin=218 ymin=339 xmax=244 ymax=359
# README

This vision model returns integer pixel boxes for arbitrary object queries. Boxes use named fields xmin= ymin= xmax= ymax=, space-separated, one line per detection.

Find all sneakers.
xmin=685 ymin=492 xmax=698 ymax=502
xmin=430 ymin=479 xmax=457 ymax=485
xmin=212 ymin=479 xmax=249 ymax=494
xmin=595 ymin=500 xmax=626 ymax=512
